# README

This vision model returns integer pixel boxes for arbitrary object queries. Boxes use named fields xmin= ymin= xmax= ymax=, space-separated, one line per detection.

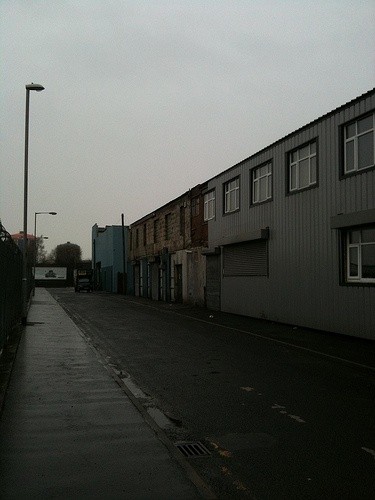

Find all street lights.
xmin=33 ymin=211 xmax=58 ymax=295
xmin=21 ymin=82 xmax=44 ymax=320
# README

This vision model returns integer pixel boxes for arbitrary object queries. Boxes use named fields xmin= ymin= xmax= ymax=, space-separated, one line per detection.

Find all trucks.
xmin=74 ymin=268 xmax=94 ymax=293
